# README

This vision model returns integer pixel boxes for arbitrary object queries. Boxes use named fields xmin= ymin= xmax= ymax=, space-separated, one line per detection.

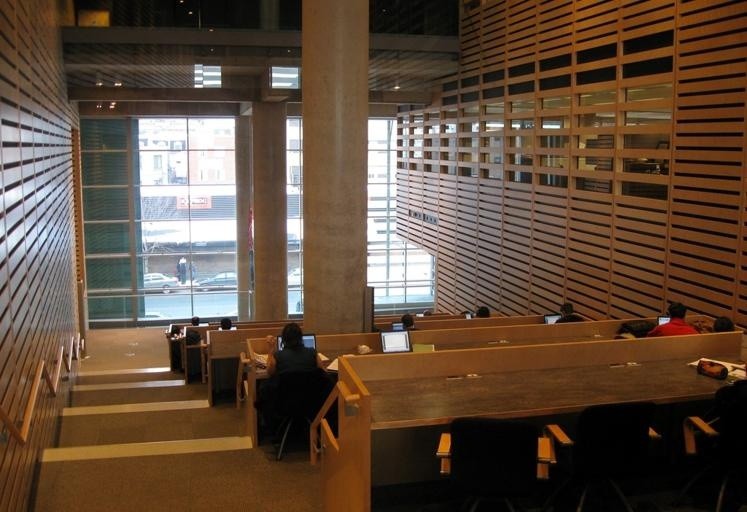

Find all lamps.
xmin=93 ymin=69 xmax=123 ymax=88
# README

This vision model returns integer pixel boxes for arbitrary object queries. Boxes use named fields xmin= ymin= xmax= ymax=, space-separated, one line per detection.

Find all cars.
xmin=144 ymin=272 xmax=179 ymax=295
xmin=194 ymin=271 xmax=237 ymax=293
xmin=288 ymin=266 xmax=303 ymax=288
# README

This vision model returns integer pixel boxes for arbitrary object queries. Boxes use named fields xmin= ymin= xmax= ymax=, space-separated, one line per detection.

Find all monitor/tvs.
xmin=217 ymin=326 xmax=237 ymax=332
xmin=656 ymin=315 xmax=672 ymax=326
xmin=392 ymin=322 xmax=415 ymax=331
xmin=196 ymin=322 xmax=210 ymax=327
xmin=276 ymin=334 xmax=317 ymax=352
xmin=380 ymin=331 xmax=411 ymax=352
xmin=543 ymin=314 xmax=562 ymax=323
xmin=415 ymin=313 xmax=424 ymax=317
xmin=464 ymin=312 xmax=474 ymax=319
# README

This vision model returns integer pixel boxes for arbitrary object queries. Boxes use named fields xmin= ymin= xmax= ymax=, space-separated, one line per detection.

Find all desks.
xmin=164 ymin=313 xmax=746 ymax=512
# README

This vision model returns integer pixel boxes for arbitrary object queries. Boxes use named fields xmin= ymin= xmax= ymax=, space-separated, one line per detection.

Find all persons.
xmin=176 ymin=255 xmax=195 ymax=283
xmin=401 ymin=314 xmax=417 ymax=331
xmin=423 ymin=310 xmax=433 ymax=316
xmin=714 ymin=316 xmax=735 ymax=332
xmin=647 ymin=302 xmax=701 ymax=336
xmin=266 ymin=322 xmax=328 ymax=377
xmin=220 ymin=318 xmax=232 ymax=330
xmin=476 ymin=306 xmax=491 ymax=318
xmin=191 ymin=317 xmax=200 ymax=326
xmin=555 ymin=303 xmax=583 ymax=321
